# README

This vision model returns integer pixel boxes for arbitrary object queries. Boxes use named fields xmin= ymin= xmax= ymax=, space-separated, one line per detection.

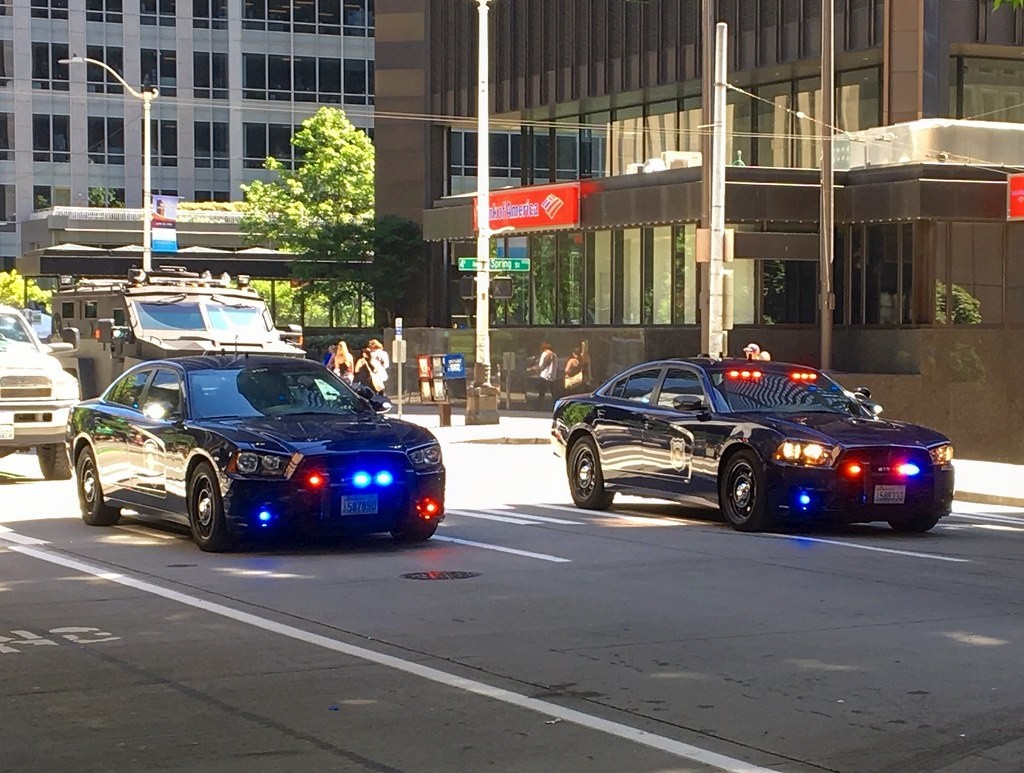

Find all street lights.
xmin=58 ymin=58 xmax=159 ymax=274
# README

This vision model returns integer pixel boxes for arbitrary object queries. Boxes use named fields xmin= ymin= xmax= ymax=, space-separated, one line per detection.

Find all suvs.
xmin=0 ymin=306 xmax=81 ymax=480
xmin=50 ymin=265 xmax=306 ymax=414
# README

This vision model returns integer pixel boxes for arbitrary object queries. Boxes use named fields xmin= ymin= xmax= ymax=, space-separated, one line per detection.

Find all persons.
xmin=325 ymin=339 xmax=389 ymax=417
xmin=743 ymin=343 xmax=760 ymax=359
xmin=526 ymin=339 xmax=592 ymax=411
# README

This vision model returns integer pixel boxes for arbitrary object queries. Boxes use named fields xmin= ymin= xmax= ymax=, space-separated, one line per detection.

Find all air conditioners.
xmin=662 ymin=151 xmax=703 ymax=172
xmin=625 ymin=163 xmax=644 ymax=173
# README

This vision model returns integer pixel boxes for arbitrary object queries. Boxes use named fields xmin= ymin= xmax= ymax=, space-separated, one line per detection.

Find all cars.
xmin=552 ymin=357 xmax=955 ymax=530
xmin=66 ymin=352 xmax=447 ymax=550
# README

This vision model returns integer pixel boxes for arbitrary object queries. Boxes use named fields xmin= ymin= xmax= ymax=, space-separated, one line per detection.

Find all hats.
xmin=743 ymin=343 xmax=760 ymax=354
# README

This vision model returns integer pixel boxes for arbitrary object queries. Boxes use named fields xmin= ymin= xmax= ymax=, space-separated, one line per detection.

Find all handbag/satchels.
xmin=564 ymin=369 xmax=583 ymax=391
xmin=370 ymin=373 xmax=385 ymax=392
